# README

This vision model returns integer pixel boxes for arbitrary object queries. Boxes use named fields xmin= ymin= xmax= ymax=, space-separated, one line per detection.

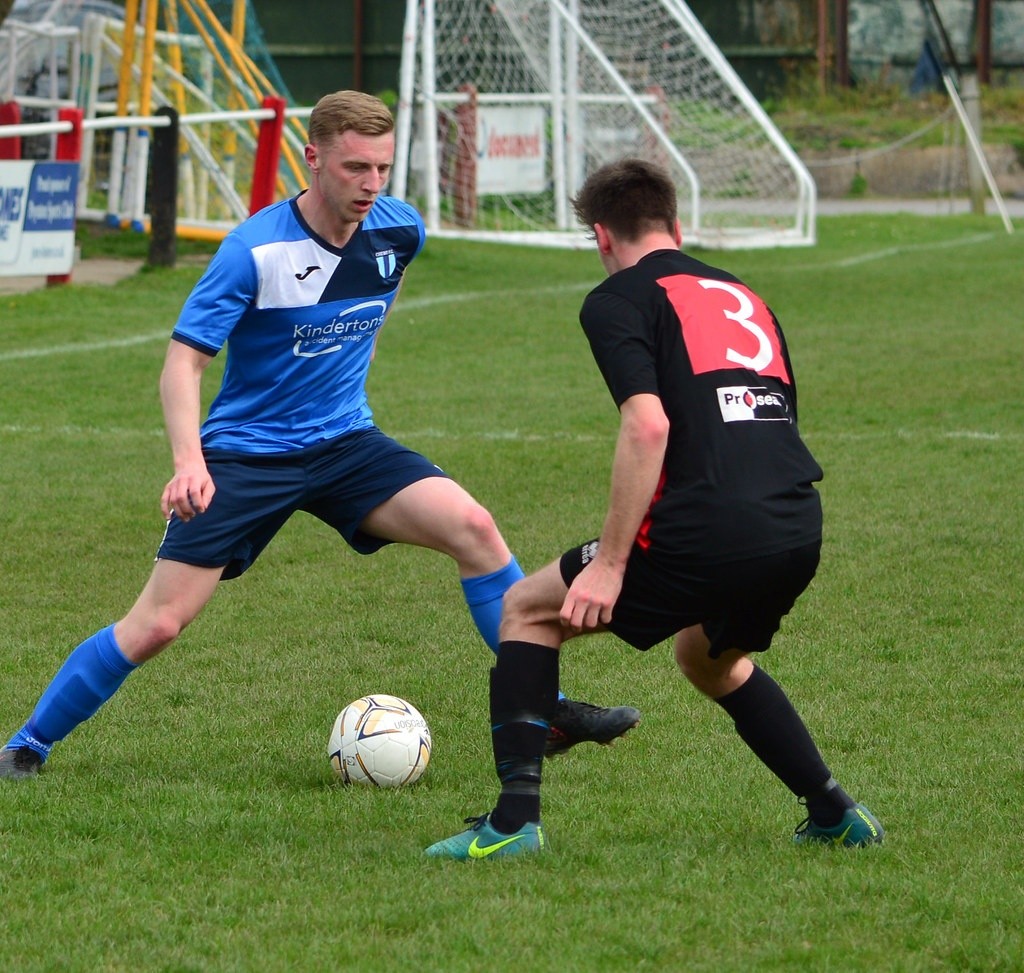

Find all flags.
xmin=909 ymin=42 xmax=947 ymax=95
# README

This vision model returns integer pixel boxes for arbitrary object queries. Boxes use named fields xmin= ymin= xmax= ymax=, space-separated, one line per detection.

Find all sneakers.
xmin=421 ymin=813 xmax=546 ymax=864
xmin=543 ymin=697 xmax=642 ymax=758
xmin=793 ymin=792 xmax=884 ymax=850
xmin=0 ymin=745 xmax=44 ymax=780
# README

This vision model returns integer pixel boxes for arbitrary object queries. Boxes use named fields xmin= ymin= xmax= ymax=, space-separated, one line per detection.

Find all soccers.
xmin=326 ymin=694 xmax=432 ymax=790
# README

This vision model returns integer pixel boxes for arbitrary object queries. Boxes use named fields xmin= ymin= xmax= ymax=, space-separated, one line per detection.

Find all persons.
xmin=423 ymin=161 xmax=884 ymax=860
xmin=0 ymin=90 xmax=640 ymax=779
